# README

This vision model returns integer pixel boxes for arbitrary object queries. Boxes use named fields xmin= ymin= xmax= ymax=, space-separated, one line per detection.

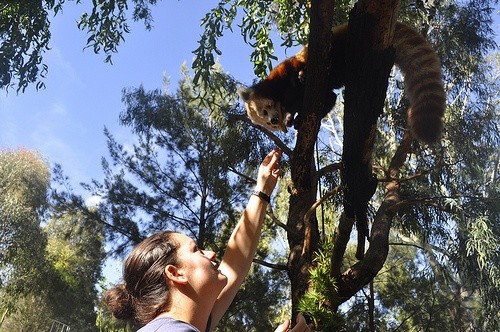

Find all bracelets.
xmin=250 ymin=189 xmax=270 ymax=204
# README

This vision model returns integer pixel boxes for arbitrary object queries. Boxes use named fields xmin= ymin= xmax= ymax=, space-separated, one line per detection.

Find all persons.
xmin=106 ymin=145 xmax=312 ymax=332
